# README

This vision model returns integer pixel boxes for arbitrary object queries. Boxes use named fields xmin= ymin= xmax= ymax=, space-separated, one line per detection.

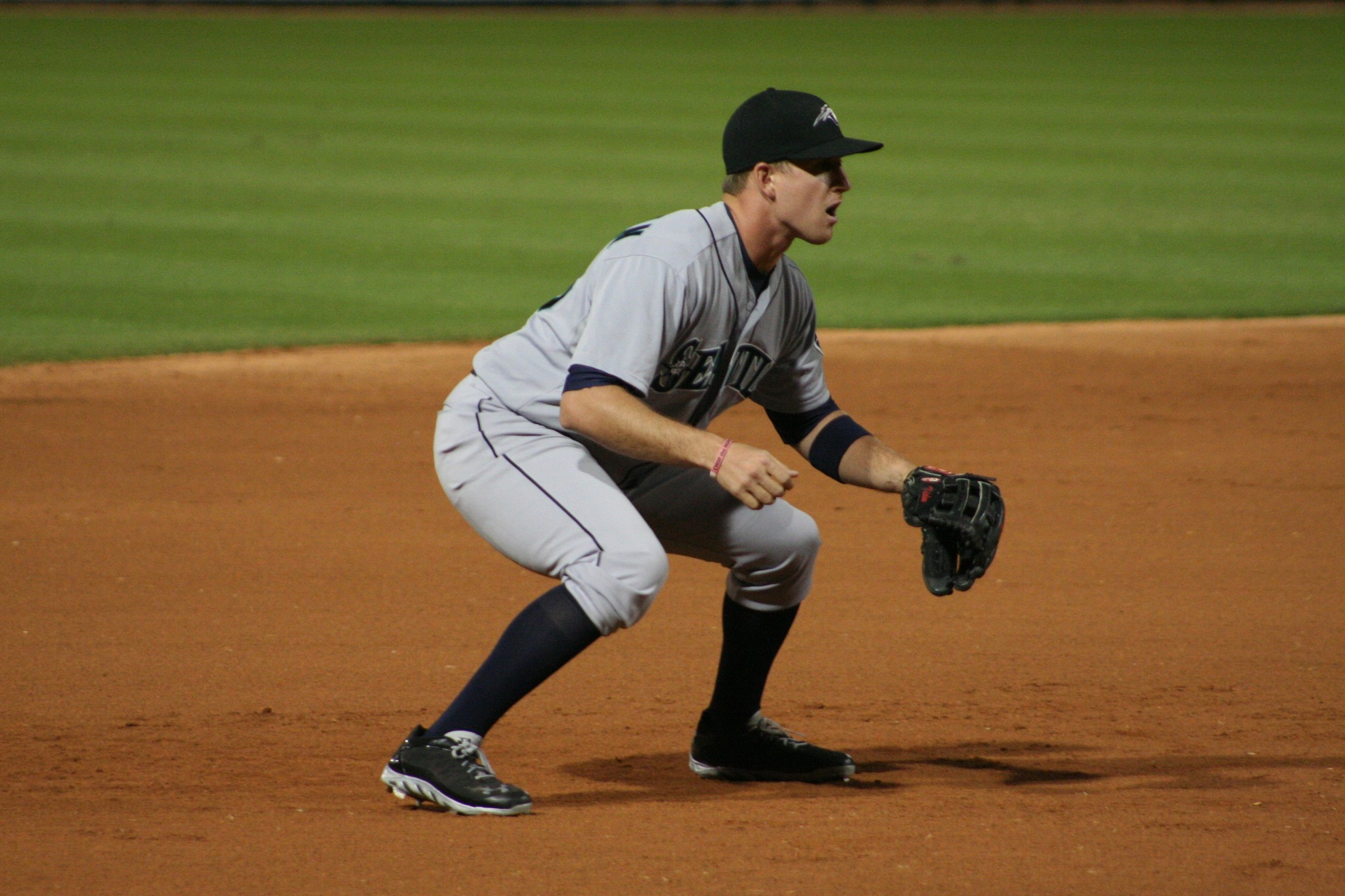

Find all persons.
xmin=381 ymin=86 xmax=1005 ymax=815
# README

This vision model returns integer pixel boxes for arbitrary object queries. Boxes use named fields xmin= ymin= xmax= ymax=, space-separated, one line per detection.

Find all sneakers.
xmin=381 ymin=724 xmax=533 ymax=816
xmin=689 ymin=705 xmax=856 ymax=782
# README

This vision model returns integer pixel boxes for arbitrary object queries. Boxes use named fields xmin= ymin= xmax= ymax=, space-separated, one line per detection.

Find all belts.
xmin=470 ymin=369 xmax=476 ymax=376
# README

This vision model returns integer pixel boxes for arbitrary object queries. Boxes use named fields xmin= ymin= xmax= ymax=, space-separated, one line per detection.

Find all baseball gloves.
xmin=902 ymin=464 xmax=1007 ymax=597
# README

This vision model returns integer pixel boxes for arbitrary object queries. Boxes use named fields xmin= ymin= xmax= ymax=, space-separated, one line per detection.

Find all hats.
xmin=721 ymin=86 xmax=885 ymax=175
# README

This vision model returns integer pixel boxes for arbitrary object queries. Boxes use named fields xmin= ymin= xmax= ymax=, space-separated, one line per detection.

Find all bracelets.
xmin=709 ymin=438 xmax=734 ymax=479
